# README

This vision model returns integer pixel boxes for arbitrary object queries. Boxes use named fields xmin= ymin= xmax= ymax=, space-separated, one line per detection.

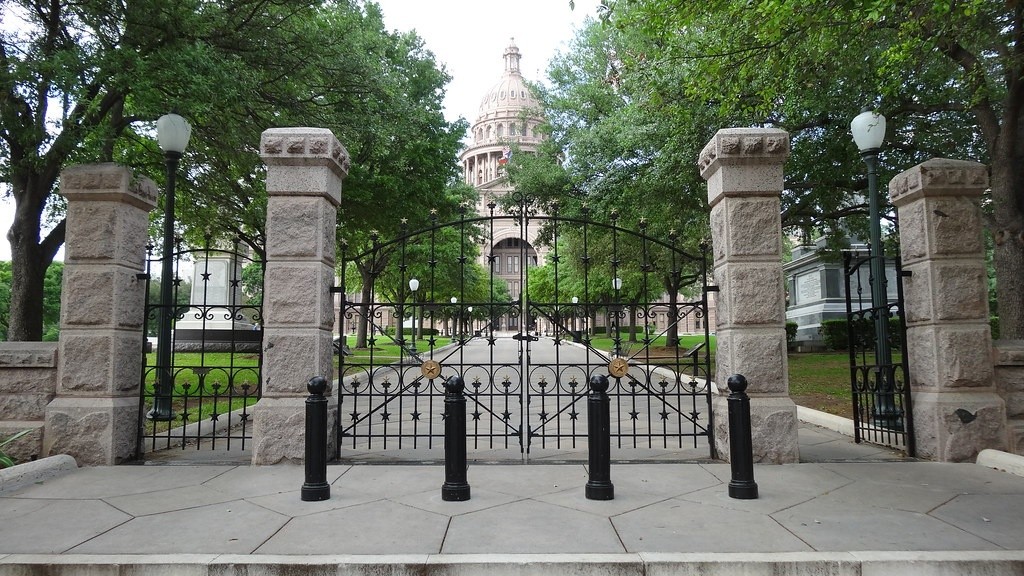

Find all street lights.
xmin=409 ymin=276 xmax=419 ymax=353
xmin=146 ymin=109 xmax=191 ymax=421
xmin=450 ymin=297 xmax=472 ymax=335
xmin=850 ymin=103 xmax=903 ymax=430
xmin=572 ymin=277 xmax=622 ymax=355
